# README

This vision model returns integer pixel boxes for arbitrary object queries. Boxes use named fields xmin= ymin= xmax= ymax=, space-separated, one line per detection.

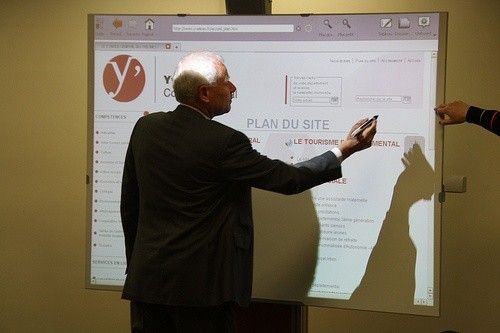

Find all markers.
xmin=352 ymin=114 xmax=380 ymax=139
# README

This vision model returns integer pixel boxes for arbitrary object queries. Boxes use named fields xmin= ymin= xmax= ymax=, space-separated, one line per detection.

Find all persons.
xmin=120 ymin=52 xmax=377 ymax=333
xmin=434 ymin=101 xmax=500 ymax=136
